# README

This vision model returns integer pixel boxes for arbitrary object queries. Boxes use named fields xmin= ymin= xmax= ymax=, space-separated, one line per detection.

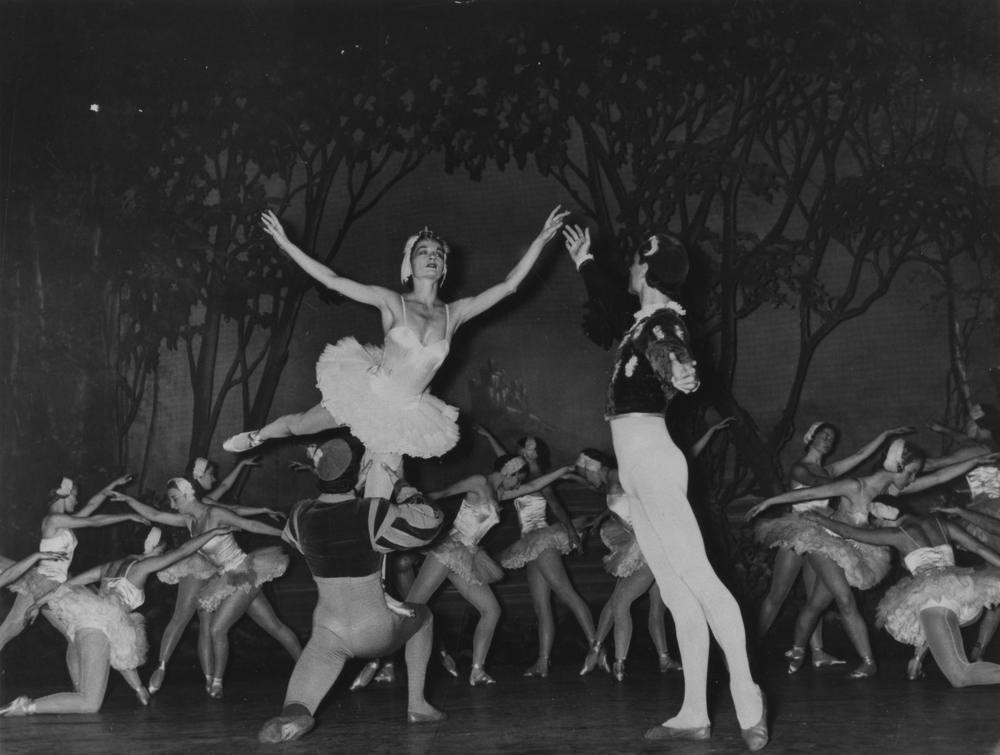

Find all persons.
xmin=0 ymin=407 xmax=1000 ymax=743
xmin=222 ymin=204 xmax=571 ymax=580
xmin=561 ymin=221 xmax=771 ymax=753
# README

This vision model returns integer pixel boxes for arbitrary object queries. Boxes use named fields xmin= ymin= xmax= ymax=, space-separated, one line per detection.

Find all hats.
xmin=311 ymin=438 xmax=351 ymax=481
